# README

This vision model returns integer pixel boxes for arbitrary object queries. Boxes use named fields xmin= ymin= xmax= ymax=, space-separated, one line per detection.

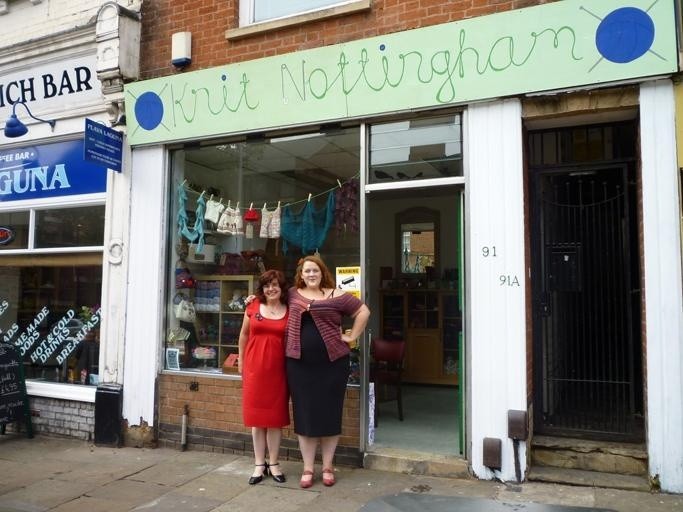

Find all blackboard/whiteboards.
xmin=0 ymin=341 xmax=30 ymax=425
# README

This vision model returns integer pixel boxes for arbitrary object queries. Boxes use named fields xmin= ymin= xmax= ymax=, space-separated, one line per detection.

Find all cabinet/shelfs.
xmin=188 ymin=273 xmax=260 ymax=374
xmin=377 ymin=287 xmax=461 ymax=386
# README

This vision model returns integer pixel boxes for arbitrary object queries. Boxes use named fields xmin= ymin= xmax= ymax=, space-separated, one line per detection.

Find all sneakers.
xmin=323 ymin=467 xmax=335 ymax=486
xmin=300 ymin=470 xmax=315 ymax=488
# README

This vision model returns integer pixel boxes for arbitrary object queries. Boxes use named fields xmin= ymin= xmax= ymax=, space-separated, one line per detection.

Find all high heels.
xmin=249 ymin=460 xmax=268 ymax=485
xmin=268 ymin=463 xmax=285 ymax=483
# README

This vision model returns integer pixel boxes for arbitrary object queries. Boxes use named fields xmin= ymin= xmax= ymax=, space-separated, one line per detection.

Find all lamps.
xmin=170 ymin=32 xmax=190 ymax=68
xmin=3 ymin=101 xmax=55 ymax=139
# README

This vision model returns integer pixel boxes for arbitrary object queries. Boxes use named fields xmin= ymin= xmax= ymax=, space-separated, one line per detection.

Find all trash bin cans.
xmin=93 ymin=383 xmax=123 ymax=448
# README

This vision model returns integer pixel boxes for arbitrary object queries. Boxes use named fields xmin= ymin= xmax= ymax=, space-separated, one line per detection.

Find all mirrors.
xmin=395 ymin=206 xmax=440 ymax=279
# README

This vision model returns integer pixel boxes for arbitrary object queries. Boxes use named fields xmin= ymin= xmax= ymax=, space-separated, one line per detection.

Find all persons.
xmin=243 ymin=256 xmax=371 ymax=489
xmin=237 ymin=269 xmax=290 ymax=485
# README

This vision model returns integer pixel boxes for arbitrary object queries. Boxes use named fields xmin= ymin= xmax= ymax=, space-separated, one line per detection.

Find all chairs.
xmin=352 ymin=339 xmax=406 ymax=428
